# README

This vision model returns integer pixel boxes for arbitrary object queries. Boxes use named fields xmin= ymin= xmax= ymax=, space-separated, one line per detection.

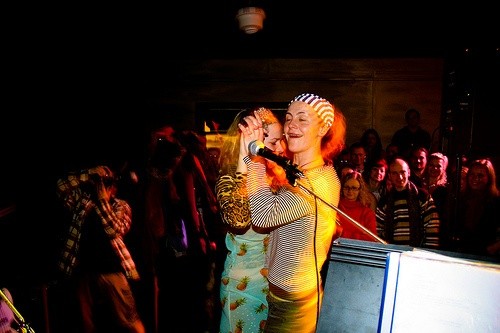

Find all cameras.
xmin=96 ymin=173 xmax=114 ymax=188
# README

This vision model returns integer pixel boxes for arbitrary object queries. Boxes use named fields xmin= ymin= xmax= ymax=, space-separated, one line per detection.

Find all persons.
xmin=0 ymin=287 xmax=20 ymax=333
xmin=216 ymin=106 xmax=289 ymax=333
xmin=238 ymin=94 xmax=347 ymax=333
xmin=331 ymin=110 xmax=500 ymax=261
xmin=56 ymin=126 xmax=230 ymax=333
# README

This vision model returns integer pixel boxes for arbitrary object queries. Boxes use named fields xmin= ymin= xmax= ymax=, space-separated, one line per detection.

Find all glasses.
xmin=466 ymin=171 xmax=485 ymax=179
xmin=342 ymin=186 xmax=359 ymax=191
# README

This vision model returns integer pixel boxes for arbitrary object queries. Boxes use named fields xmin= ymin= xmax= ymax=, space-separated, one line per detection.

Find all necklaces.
xmin=297 ymin=157 xmax=318 ymax=169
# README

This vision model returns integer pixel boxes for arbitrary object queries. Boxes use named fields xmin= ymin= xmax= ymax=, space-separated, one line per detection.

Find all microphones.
xmin=248 ymin=139 xmax=305 ymax=178
